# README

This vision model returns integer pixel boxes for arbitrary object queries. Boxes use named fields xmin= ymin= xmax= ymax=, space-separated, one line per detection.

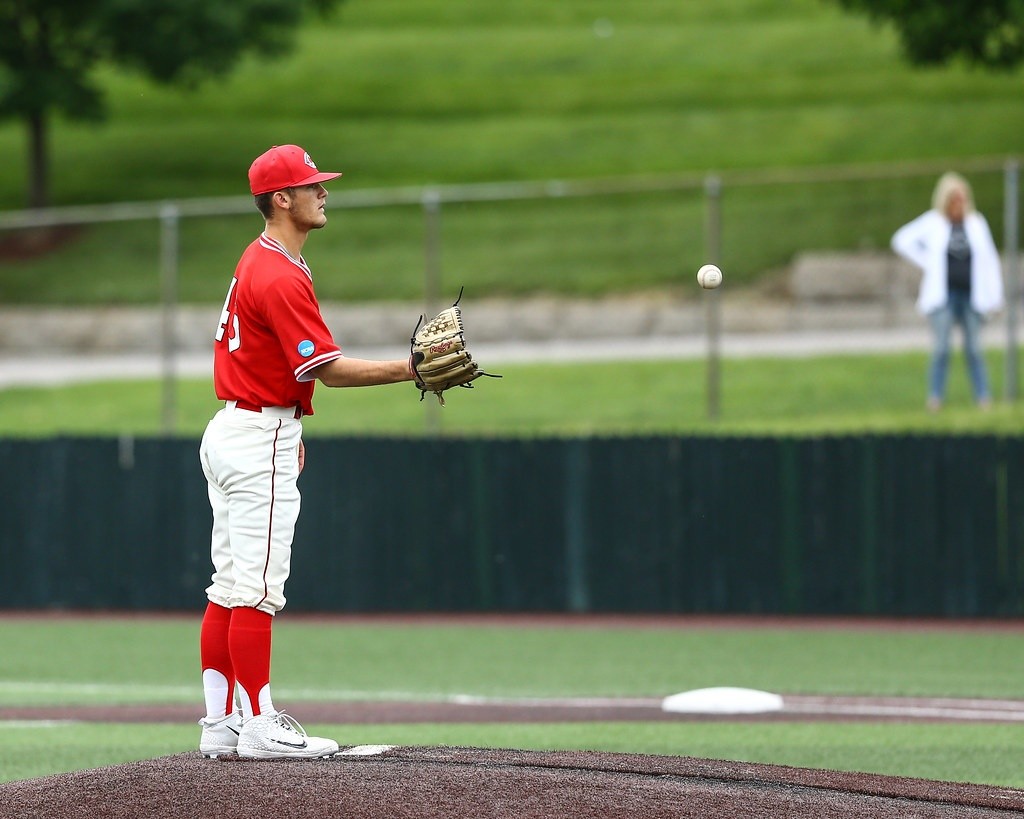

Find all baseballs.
xmin=696 ymin=265 xmax=723 ymax=289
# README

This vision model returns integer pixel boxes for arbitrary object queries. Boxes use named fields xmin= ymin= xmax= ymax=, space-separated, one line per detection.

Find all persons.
xmin=888 ymin=171 xmax=1006 ymax=412
xmin=199 ymin=143 xmax=483 ymax=759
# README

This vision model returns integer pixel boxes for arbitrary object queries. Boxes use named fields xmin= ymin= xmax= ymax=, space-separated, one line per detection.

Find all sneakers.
xmin=199 ymin=712 xmax=244 ymax=758
xmin=235 ymin=710 xmax=339 ymax=759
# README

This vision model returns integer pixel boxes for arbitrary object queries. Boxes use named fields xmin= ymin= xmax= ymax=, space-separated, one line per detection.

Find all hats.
xmin=248 ymin=145 xmax=343 ymax=196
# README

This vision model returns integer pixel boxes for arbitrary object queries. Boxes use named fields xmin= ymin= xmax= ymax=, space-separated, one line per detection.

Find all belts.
xmin=235 ymin=399 xmax=300 ymax=419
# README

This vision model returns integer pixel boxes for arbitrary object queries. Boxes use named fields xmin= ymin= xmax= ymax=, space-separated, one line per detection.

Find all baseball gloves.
xmin=410 ymin=306 xmax=485 ymax=391
